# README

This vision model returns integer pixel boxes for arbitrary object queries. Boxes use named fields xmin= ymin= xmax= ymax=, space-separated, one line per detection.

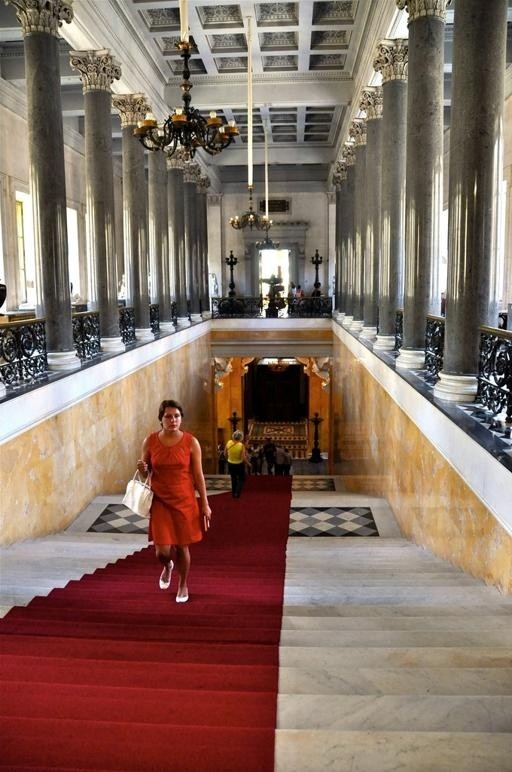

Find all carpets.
xmin=87 ymin=502 xmax=379 ymax=537
xmin=193 ymin=477 xmax=336 ymax=491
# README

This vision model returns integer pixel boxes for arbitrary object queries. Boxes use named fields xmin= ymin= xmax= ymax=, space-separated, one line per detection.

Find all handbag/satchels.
xmin=122 ymin=469 xmax=153 ymax=518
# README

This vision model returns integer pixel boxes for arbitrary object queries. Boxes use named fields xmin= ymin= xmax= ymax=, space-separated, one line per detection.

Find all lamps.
xmin=132 ymin=0 xmax=280 ymax=248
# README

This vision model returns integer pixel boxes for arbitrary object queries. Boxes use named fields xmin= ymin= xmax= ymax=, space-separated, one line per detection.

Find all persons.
xmin=441 ymin=291 xmax=446 ymax=317
xmin=224 ymin=429 xmax=252 ymax=498
xmin=217 ymin=436 xmax=293 ymax=478
xmin=135 ymin=398 xmax=213 ymax=604
xmin=287 ymin=281 xmax=326 ymax=316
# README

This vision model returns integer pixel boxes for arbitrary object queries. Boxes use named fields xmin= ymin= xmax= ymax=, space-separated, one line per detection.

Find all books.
xmin=203 ymin=514 xmax=211 ymax=532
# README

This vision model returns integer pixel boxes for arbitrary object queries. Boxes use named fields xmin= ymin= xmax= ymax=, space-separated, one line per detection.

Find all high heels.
xmin=159 ymin=560 xmax=174 ymax=590
xmin=176 ymin=588 xmax=188 ymax=603
